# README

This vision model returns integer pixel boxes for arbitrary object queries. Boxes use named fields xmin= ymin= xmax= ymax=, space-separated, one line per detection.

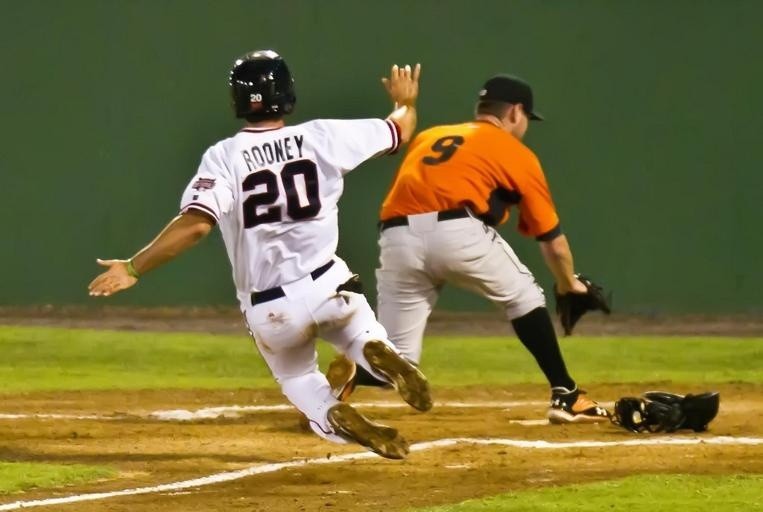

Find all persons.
xmin=297 ymin=73 xmax=615 ymax=425
xmin=85 ymin=50 xmax=434 ymax=461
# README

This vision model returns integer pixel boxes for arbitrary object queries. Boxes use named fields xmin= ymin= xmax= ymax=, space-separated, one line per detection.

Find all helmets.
xmin=640 ymin=389 xmax=719 ymax=432
xmin=231 ymin=50 xmax=295 ymax=117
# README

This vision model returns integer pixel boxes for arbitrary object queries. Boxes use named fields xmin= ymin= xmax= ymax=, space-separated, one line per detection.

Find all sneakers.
xmin=300 ymin=357 xmax=358 ymax=428
xmin=548 ymin=384 xmax=612 ymax=424
xmin=327 ymin=402 xmax=410 ymax=460
xmin=364 ymin=340 xmax=432 ymax=411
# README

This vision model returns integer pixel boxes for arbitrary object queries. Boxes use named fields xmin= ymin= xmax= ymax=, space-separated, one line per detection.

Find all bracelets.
xmin=124 ymin=258 xmax=142 ymax=279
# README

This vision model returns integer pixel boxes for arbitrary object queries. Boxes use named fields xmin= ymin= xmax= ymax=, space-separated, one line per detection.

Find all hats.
xmin=480 ymin=77 xmax=543 ymax=121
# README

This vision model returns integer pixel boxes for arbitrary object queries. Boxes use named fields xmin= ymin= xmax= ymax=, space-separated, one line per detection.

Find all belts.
xmin=251 ymin=259 xmax=335 ymax=306
xmin=381 ymin=207 xmax=470 ymax=230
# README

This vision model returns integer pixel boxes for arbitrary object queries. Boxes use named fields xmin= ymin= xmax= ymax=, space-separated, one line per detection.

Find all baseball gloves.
xmin=554 ymin=272 xmax=610 ymax=335
